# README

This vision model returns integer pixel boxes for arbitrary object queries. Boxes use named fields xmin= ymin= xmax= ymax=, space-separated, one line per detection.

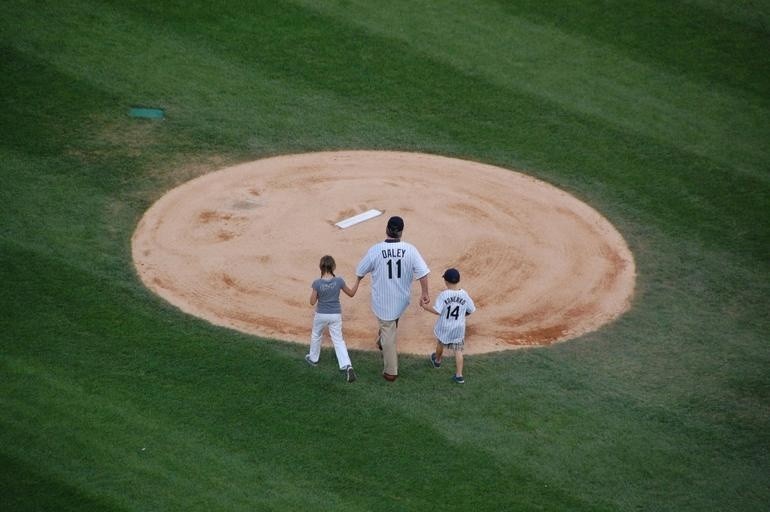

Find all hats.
xmin=387 ymin=216 xmax=403 ymax=235
xmin=319 ymin=254 xmax=336 ymax=272
xmin=442 ymin=268 xmax=460 ymax=283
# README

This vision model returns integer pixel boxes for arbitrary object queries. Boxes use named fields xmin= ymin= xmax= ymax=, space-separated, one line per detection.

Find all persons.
xmin=421 ymin=269 xmax=475 ymax=384
xmin=356 ymin=216 xmax=430 ymax=381
xmin=305 ymin=255 xmax=363 ymax=382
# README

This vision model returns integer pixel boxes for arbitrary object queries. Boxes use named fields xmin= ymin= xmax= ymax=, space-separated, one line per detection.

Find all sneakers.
xmin=382 ymin=371 xmax=395 ymax=382
xmin=305 ymin=354 xmax=318 ymax=366
xmin=452 ymin=374 xmax=464 ymax=384
xmin=376 ymin=337 xmax=383 ymax=351
xmin=430 ymin=352 xmax=441 ymax=369
xmin=345 ymin=366 xmax=356 ymax=382
